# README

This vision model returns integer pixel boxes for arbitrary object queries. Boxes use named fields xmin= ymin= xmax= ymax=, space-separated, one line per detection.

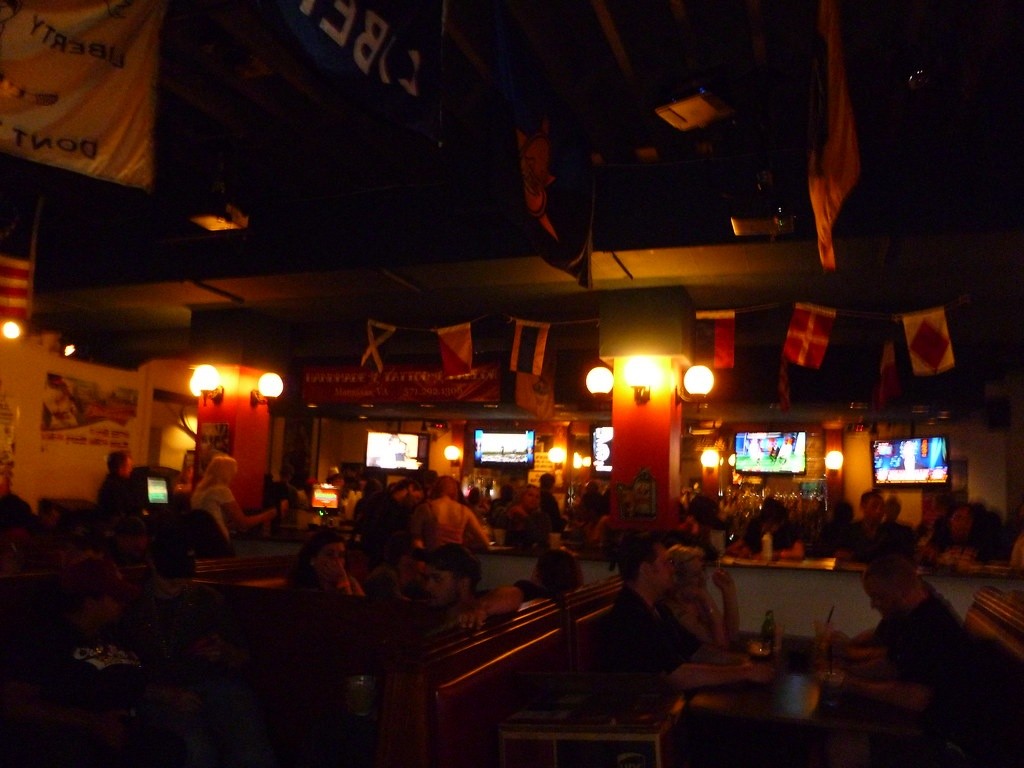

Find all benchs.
xmin=372 ymin=573 xmax=625 ymax=768
xmin=871 ymin=587 xmax=1024 ymax=768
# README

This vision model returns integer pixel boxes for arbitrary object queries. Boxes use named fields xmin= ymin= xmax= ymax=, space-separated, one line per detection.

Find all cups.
xmin=761 ymin=610 xmax=774 ymax=637
xmin=494 ymin=528 xmax=506 ymax=544
xmin=747 ymin=637 xmax=774 ymax=662
xmin=548 ymin=532 xmax=561 ymax=549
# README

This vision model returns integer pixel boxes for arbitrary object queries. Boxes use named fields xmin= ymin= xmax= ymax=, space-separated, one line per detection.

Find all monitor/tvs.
xmin=475 ymin=429 xmax=535 ymax=468
xmin=590 ymin=426 xmax=612 ymax=478
xmin=870 ymin=434 xmax=951 ymax=490
xmin=735 ymin=430 xmax=807 ymax=477
xmin=147 ymin=477 xmax=169 ymax=504
xmin=365 ymin=431 xmax=429 ymax=471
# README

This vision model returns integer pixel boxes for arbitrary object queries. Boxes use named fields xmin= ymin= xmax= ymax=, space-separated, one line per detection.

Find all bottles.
xmin=725 ymin=479 xmax=825 ymax=520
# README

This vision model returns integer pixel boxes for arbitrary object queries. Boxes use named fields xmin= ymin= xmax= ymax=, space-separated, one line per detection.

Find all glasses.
xmin=414 ymin=571 xmax=455 ymax=586
xmin=646 ymin=557 xmax=677 ymax=567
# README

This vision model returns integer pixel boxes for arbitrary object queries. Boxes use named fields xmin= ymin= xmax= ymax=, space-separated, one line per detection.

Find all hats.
xmin=152 ymin=526 xmax=195 ymax=579
xmin=411 ymin=543 xmax=490 ymax=582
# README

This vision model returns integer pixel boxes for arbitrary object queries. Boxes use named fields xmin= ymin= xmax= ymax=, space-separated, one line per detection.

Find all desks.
xmin=687 ymin=632 xmax=926 ymax=768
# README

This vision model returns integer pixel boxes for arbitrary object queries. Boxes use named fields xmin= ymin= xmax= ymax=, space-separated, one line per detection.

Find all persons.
xmin=678 ymin=487 xmax=1024 ymax=569
xmin=26 ymin=559 xmax=275 ymax=768
xmin=825 ymin=524 xmax=990 ymax=768
xmin=1 ymin=452 xmax=314 ymax=572
xmin=602 ymin=536 xmax=777 ymax=768
xmin=299 ymin=461 xmax=609 ymax=632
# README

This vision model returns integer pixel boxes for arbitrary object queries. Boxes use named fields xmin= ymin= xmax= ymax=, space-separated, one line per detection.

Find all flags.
xmin=259 ymin=0 xmax=446 ymax=146
xmin=902 ymin=307 xmax=955 ymax=376
xmin=781 ymin=305 xmax=836 ymax=368
xmin=806 ymin=0 xmax=861 ymax=274
xmin=509 ymin=319 xmax=550 ymax=376
xmin=514 ymin=354 xmax=555 ymax=416
xmin=0 ymin=0 xmax=163 ymax=189
xmin=879 ymin=341 xmax=901 ymax=409
xmin=496 ymin=4 xmax=598 ymax=291
xmin=694 ymin=310 xmax=735 ymax=368
xmin=438 ymin=322 xmax=473 ymax=381
xmin=358 ymin=321 xmax=397 ymax=372
